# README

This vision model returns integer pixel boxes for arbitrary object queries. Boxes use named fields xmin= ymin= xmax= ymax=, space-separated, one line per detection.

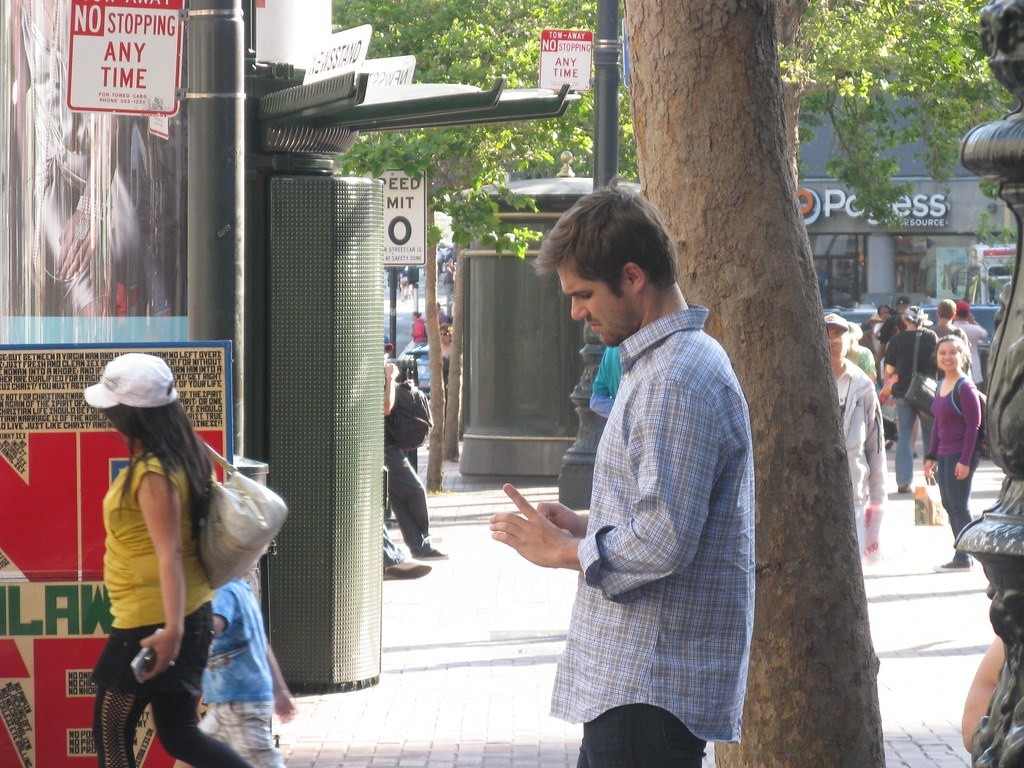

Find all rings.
xmin=169 ymin=661 xmax=175 ymax=665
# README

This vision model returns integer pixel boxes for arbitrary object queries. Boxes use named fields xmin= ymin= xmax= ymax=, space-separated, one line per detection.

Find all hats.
xmin=896 ymin=295 xmax=912 ymax=305
xmin=384 ymin=335 xmax=393 ymax=352
xmin=825 ymin=312 xmax=848 ymax=331
xmin=956 ymin=300 xmax=970 ymax=316
xmin=83 ymin=353 xmax=178 ymax=409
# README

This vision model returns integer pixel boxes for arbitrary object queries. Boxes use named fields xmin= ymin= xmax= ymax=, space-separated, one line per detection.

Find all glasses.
xmin=440 ymin=332 xmax=450 ymax=337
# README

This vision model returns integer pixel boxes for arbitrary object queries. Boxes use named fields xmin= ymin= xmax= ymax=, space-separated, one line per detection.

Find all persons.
xmin=382 ymin=343 xmax=448 ymax=579
xmin=440 ymin=322 xmax=452 ymax=390
xmin=962 ymin=636 xmax=1005 ymax=754
xmin=411 ymin=311 xmax=426 ymax=343
xmin=399 ymin=265 xmax=408 ymax=302
xmin=171 ymin=577 xmax=298 ymax=768
xmin=437 ymin=247 xmax=456 ymax=302
xmin=825 ymin=313 xmax=886 ymax=557
xmin=590 ymin=346 xmax=622 ymax=420
xmin=886 ymin=306 xmax=944 ymax=492
xmin=490 ymin=188 xmax=756 ymax=768
xmin=924 ymin=334 xmax=982 ymax=572
xmin=437 ymin=302 xmax=448 ymax=325
xmin=845 ymin=295 xmax=919 ymax=456
xmin=929 ymin=299 xmax=987 ymax=388
xmin=85 ymin=353 xmax=256 ymax=768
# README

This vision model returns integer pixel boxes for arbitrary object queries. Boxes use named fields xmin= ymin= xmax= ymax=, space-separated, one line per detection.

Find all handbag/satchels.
xmin=197 ymin=439 xmax=289 ymax=590
xmin=914 ymin=471 xmax=946 ymax=527
xmin=905 ymin=372 xmax=938 ymax=420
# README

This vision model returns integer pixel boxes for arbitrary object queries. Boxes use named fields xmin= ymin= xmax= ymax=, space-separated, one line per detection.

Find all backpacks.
xmin=935 ymin=377 xmax=990 ymax=459
xmin=385 ymin=381 xmax=434 ymax=452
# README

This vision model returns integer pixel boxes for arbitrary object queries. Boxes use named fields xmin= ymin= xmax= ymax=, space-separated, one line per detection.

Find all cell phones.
xmin=130 ymin=628 xmax=164 ymax=683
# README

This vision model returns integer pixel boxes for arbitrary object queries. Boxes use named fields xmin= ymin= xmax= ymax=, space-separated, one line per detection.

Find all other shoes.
xmin=897 ymin=484 xmax=912 ymax=493
xmin=383 ymin=562 xmax=432 ymax=581
xmin=412 ymin=546 xmax=448 ymax=560
xmin=914 ymin=451 xmax=919 ymax=458
xmin=933 ymin=559 xmax=973 ymax=572
xmin=886 ymin=440 xmax=894 ymax=449
